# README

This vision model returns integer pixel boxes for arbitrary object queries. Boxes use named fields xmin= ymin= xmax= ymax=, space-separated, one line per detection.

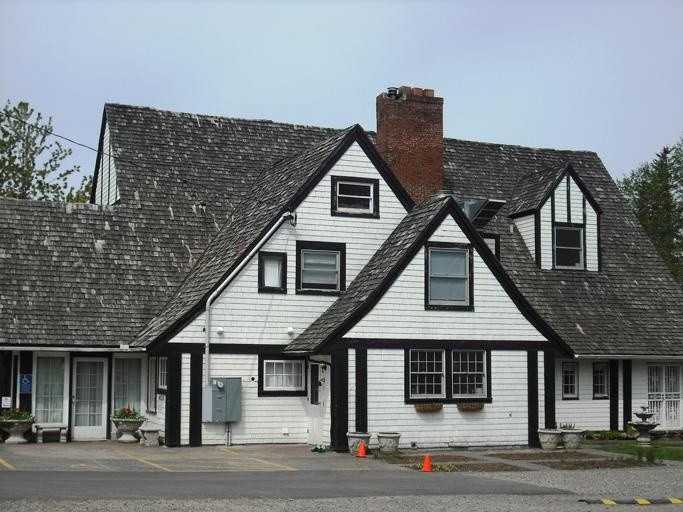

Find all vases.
xmin=0 ymin=419 xmax=32 ymax=443
xmin=109 ymin=417 xmax=147 ymax=444
xmin=345 ymin=429 xmax=400 ymax=456
xmin=536 ymin=428 xmax=585 ymax=451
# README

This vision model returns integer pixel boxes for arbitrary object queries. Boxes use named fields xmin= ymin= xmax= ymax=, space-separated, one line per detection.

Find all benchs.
xmin=34 ymin=423 xmax=67 ymax=443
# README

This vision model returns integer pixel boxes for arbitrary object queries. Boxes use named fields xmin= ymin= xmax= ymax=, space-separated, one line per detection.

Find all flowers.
xmin=0 ymin=406 xmax=34 ymax=421
xmin=109 ymin=407 xmax=145 ymax=420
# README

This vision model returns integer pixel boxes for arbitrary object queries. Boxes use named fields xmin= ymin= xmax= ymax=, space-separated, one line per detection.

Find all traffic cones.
xmin=356 ymin=443 xmax=367 ymax=458
xmin=422 ymin=454 xmax=433 ymax=472
